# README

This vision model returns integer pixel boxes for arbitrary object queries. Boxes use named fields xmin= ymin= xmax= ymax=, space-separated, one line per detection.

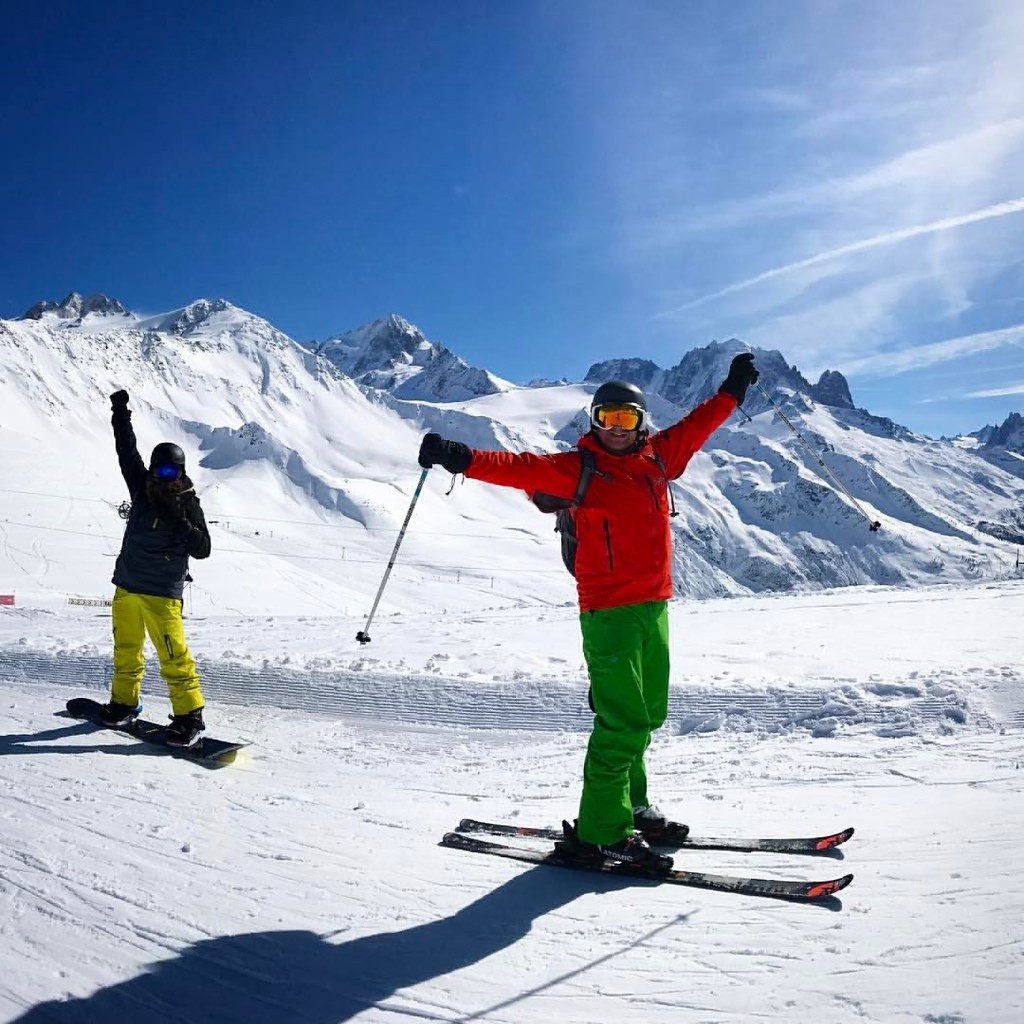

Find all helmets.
xmin=149 ymin=443 xmax=185 ymax=467
xmin=590 ymin=380 xmax=648 ymax=410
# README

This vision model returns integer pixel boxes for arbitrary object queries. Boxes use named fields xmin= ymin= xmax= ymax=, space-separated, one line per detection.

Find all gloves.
xmin=157 ymin=507 xmax=186 ymax=529
xmin=718 ymin=354 xmax=760 ymax=406
xmin=418 ymin=433 xmax=464 ymax=469
xmin=109 ymin=390 xmax=129 ymax=411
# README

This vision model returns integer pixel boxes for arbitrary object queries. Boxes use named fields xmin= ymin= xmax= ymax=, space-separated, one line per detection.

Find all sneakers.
xmin=167 ymin=710 xmax=205 ymax=746
xmin=634 ymin=804 xmax=689 ymax=845
xmin=568 ymin=832 xmax=673 ymax=879
xmin=102 ymin=708 xmax=139 ymax=726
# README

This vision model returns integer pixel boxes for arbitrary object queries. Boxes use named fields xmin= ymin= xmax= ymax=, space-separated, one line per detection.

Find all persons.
xmin=102 ymin=389 xmax=211 ymax=747
xmin=418 ymin=354 xmax=760 ymax=880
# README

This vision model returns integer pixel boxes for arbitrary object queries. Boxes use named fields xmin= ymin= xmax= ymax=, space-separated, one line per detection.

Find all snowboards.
xmin=64 ymin=696 xmax=255 ymax=761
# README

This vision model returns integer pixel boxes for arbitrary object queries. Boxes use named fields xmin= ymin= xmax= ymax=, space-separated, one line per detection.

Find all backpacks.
xmin=557 ymin=450 xmax=666 ymax=576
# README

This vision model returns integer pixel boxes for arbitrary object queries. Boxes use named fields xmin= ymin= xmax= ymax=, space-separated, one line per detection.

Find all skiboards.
xmin=443 ymin=816 xmax=859 ymax=902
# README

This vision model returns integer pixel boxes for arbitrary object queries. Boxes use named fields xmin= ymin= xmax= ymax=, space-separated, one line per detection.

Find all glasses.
xmin=152 ymin=464 xmax=182 ymax=480
xmin=592 ymin=403 xmax=647 ymax=434
